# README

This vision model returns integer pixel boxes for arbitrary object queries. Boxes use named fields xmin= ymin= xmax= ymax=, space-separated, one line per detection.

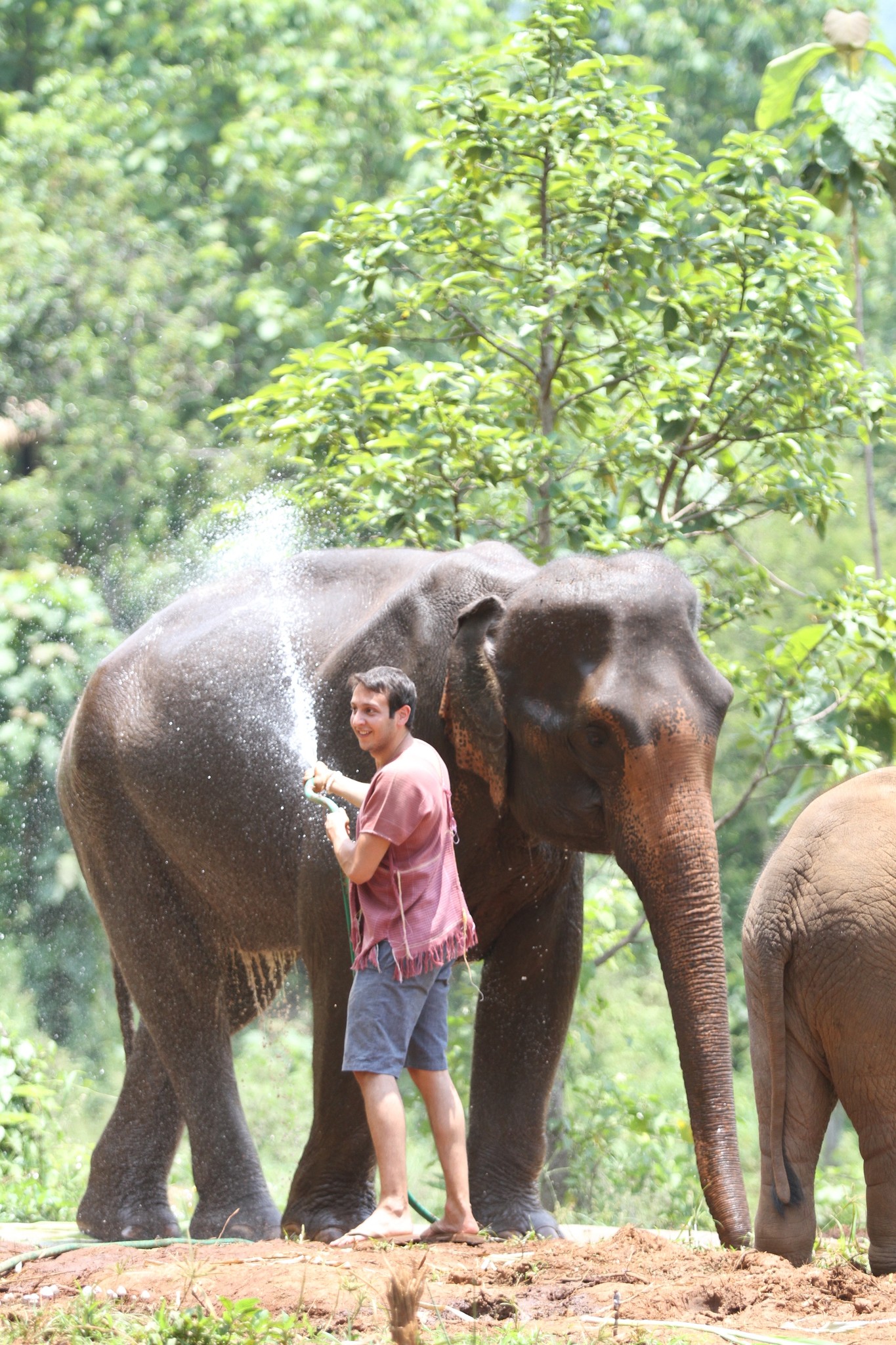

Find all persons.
xmin=303 ymin=666 xmax=485 ymax=1249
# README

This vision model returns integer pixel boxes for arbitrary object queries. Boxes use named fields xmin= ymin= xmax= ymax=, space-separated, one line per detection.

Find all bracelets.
xmin=321 ymin=770 xmax=343 ymax=796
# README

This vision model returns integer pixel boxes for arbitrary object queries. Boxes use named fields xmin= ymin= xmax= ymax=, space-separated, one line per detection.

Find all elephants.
xmin=739 ymin=765 xmax=896 ymax=1278
xmin=55 ymin=537 xmax=752 ymax=1253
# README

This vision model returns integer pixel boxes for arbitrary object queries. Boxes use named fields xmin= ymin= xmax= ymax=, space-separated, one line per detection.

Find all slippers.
xmin=328 ymin=1229 xmax=417 ymax=1251
xmin=420 ymin=1220 xmax=485 ymax=1244
